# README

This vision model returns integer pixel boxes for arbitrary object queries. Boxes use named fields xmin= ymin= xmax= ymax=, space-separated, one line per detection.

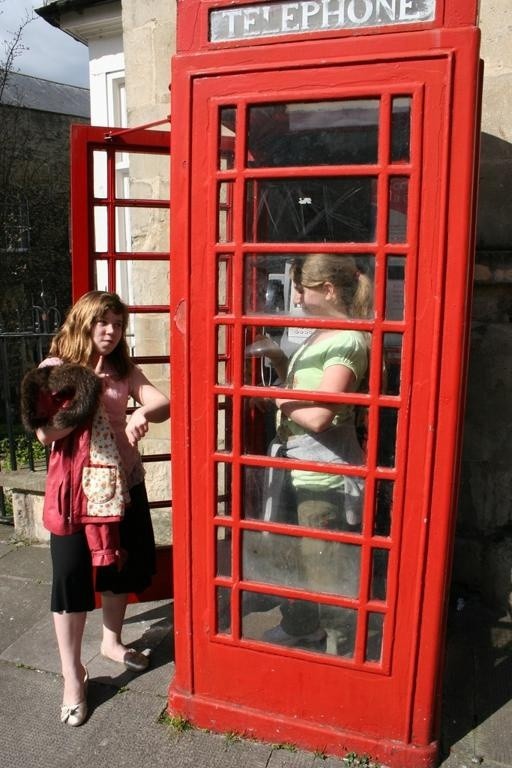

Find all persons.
xmin=19 ymin=292 xmax=169 ymax=726
xmin=259 ymin=254 xmax=387 ymax=664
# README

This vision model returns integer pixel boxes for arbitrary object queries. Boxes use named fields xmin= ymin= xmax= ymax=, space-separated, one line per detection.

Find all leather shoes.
xmin=100 ymin=641 xmax=149 ymax=670
xmin=62 ymin=665 xmax=89 ymax=727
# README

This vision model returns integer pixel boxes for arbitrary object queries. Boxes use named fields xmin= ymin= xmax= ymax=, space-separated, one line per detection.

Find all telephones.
xmin=266 ymin=273 xmax=289 ymax=343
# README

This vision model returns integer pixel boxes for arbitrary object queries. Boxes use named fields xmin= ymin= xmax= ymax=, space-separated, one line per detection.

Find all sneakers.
xmin=262 ymin=627 xmax=326 ymax=647
